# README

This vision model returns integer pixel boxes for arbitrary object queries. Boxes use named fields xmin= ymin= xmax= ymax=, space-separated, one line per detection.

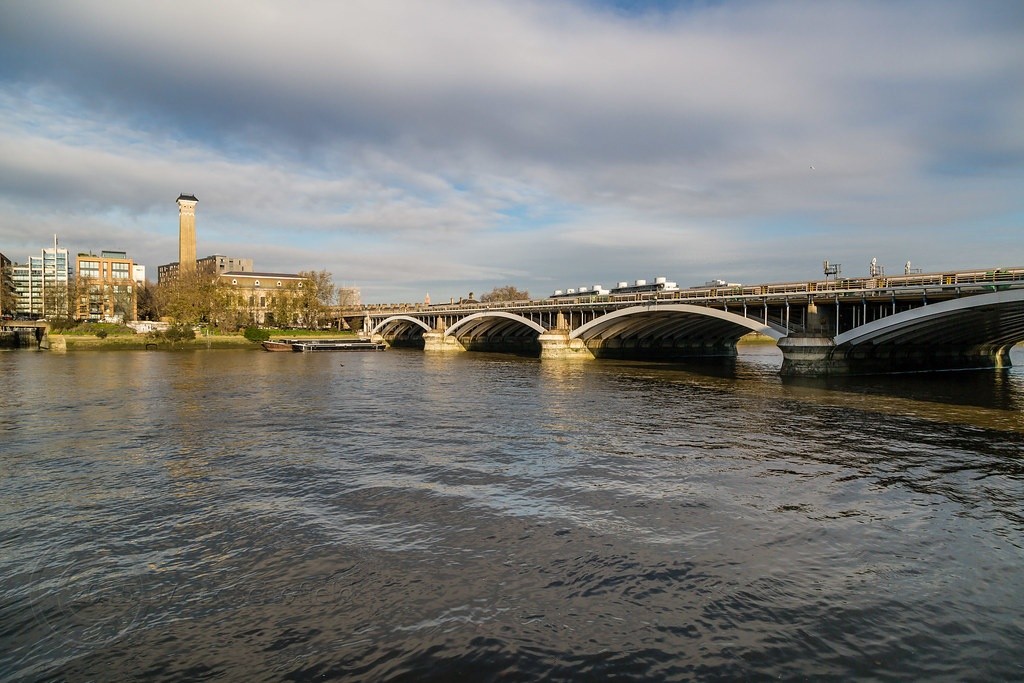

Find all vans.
xmin=97 ymin=317 xmax=114 ymax=323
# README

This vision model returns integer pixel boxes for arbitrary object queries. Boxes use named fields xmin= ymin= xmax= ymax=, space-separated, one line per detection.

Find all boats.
xmin=262 ymin=338 xmax=387 ymax=352
